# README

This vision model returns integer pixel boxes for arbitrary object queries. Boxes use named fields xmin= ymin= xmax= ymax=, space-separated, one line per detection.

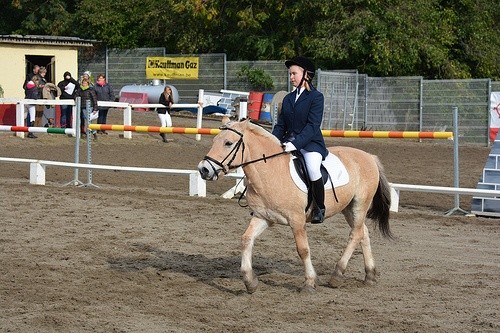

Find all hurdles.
xmin=0 ymin=124 xmax=73 ymax=136
xmin=88 ymin=121 xmax=224 ymax=135
xmin=319 ymin=130 xmax=454 ymax=140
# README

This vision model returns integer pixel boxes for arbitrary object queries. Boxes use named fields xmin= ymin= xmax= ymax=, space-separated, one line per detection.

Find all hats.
xmin=285 ymin=55 xmax=315 ymax=79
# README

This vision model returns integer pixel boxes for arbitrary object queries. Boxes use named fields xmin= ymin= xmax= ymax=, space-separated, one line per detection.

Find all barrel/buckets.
xmin=248 ymin=91 xmax=277 ymax=123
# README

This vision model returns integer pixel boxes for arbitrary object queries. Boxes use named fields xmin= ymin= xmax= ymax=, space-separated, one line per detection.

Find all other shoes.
xmin=103 ymin=131 xmax=108 ymax=133
xmin=160 ymin=132 xmax=168 ymax=142
xmin=97 ymin=130 xmax=103 ymax=133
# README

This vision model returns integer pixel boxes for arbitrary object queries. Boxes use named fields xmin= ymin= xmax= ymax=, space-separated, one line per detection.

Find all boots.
xmin=28 ymin=121 xmax=37 ymax=137
xmin=310 ymin=176 xmax=325 ymax=222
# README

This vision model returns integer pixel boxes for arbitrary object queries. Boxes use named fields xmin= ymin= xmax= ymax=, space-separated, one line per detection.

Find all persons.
xmin=272 ymin=56 xmax=327 ymax=223
xmin=22 ymin=65 xmax=48 ymax=139
xmin=157 ymin=86 xmax=174 ymax=142
xmin=57 ymin=71 xmax=115 ymax=137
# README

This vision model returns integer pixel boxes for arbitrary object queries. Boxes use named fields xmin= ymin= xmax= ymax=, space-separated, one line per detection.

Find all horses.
xmin=198 ymin=112 xmax=392 ymax=294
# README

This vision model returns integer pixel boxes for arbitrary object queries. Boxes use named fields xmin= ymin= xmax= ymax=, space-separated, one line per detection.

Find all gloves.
xmin=283 ymin=141 xmax=297 ymax=151
